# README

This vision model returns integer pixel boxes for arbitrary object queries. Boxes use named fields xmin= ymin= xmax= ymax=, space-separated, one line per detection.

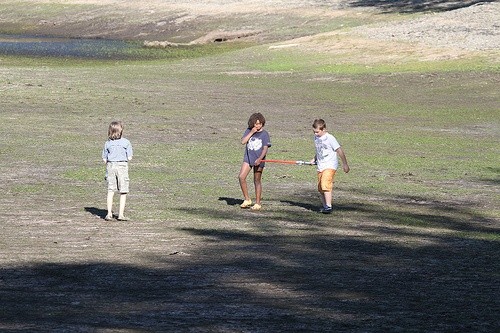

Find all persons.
xmin=310 ymin=119 xmax=350 ymax=214
xmin=102 ymin=121 xmax=134 ymax=221
xmin=237 ymin=112 xmax=271 ymax=210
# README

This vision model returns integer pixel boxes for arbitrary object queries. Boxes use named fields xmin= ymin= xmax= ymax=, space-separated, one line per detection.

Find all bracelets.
xmin=314 ymin=156 xmax=317 ymax=161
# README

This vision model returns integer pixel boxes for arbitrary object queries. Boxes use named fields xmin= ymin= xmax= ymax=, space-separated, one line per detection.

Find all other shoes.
xmin=104 ymin=215 xmax=113 ymax=221
xmin=118 ymin=214 xmax=130 ymax=221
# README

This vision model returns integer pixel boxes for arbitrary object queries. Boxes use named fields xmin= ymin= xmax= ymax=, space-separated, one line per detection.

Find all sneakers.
xmin=239 ymin=199 xmax=253 ymax=208
xmin=319 ymin=205 xmax=333 ymax=213
xmin=250 ymin=203 xmax=262 ymax=210
